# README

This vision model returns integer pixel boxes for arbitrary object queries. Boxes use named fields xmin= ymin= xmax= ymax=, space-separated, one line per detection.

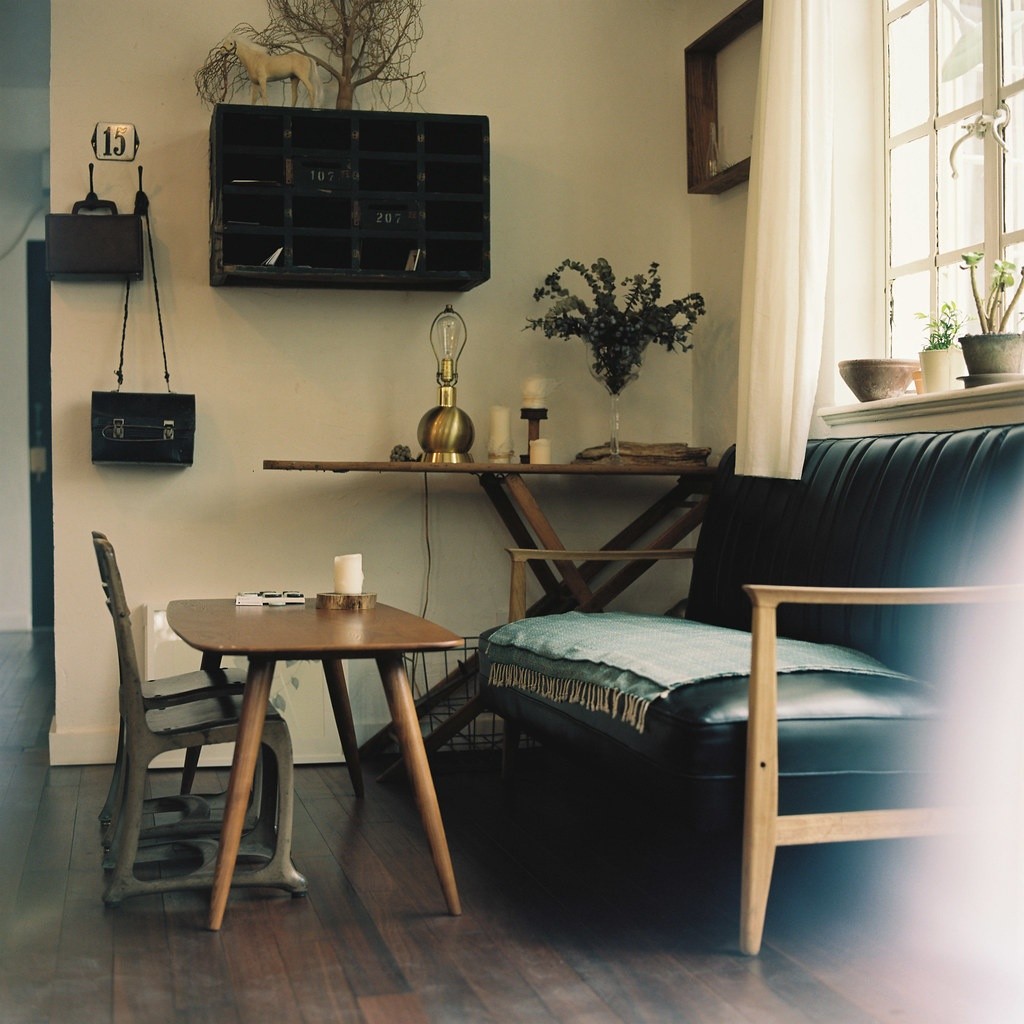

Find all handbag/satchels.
xmin=44 ymin=192 xmax=145 ymax=282
xmin=90 ymin=389 xmax=194 ymax=467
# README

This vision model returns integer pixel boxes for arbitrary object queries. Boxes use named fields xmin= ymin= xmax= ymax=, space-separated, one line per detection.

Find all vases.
xmin=839 ymin=352 xmax=919 ymax=404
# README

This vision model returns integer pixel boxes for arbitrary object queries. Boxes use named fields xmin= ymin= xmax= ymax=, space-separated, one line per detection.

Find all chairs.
xmin=91 ymin=530 xmax=310 ymax=905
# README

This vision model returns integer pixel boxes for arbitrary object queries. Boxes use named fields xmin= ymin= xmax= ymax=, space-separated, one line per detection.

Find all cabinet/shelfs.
xmin=209 ymin=103 xmax=494 ymax=290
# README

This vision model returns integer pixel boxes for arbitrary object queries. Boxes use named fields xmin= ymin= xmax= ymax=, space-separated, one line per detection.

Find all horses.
xmin=221 ymin=32 xmax=327 ymax=109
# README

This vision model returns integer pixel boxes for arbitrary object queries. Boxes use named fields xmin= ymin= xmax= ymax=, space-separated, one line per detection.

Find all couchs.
xmin=478 ymin=422 xmax=1024 ymax=955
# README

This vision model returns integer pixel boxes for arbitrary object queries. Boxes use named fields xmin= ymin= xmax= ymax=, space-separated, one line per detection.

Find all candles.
xmin=334 ymin=553 xmax=365 ymax=597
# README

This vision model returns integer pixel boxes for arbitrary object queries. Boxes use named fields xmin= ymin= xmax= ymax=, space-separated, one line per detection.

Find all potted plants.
xmin=916 ymin=300 xmax=964 ymax=394
xmin=959 ymin=250 xmax=1024 ymax=374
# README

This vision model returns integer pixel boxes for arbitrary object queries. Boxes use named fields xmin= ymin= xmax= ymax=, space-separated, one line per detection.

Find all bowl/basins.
xmin=834 ymin=357 xmax=921 ymax=403
xmin=910 ymin=370 xmax=925 ymax=395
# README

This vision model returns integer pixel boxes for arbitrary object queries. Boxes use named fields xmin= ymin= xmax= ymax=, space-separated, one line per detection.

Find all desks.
xmin=263 ymin=457 xmax=721 ymax=790
xmin=167 ymin=581 xmax=466 ymax=929
